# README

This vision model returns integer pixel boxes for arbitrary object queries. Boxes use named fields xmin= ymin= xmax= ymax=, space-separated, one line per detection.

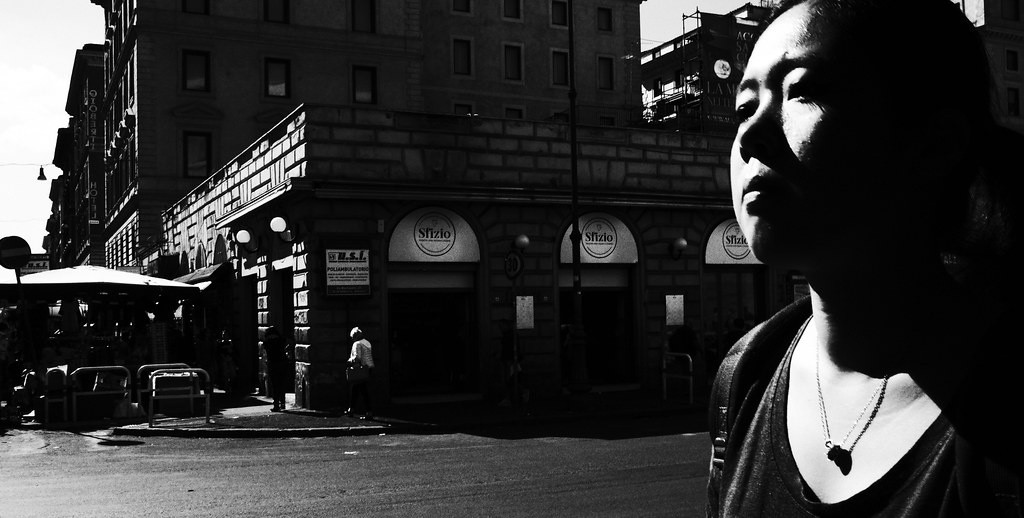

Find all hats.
xmin=350 ymin=327 xmax=362 ymax=338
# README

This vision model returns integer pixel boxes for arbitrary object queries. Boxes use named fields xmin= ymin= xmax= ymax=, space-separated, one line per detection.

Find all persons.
xmin=667 ymin=321 xmax=700 ymax=365
xmin=497 ymin=318 xmax=530 ymax=407
xmin=346 ymin=326 xmax=377 ymax=420
xmin=705 ymin=0 xmax=1024 ymax=518
xmin=259 ymin=328 xmax=291 ymax=413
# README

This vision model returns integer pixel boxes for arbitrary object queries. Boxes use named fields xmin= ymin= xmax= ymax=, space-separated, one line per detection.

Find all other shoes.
xmin=343 ymin=410 xmax=353 ymax=416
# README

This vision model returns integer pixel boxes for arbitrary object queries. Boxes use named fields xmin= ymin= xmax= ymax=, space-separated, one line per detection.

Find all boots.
xmin=270 ymin=402 xmax=280 ymax=412
xmin=280 ymin=402 xmax=285 ymax=409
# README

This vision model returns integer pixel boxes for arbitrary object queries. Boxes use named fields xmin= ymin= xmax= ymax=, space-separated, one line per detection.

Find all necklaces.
xmin=808 ymin=316 xmax=897 ymax=476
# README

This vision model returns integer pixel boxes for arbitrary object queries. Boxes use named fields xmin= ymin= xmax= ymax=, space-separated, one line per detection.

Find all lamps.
xmin=510 ymin=235 xmax=530 ymax=257
xmin=237 ymin=230 xmax=261 ymax=253
xmin=270 ymin=215 xmax=296 ymax=244
xmin=667 ymin=237 xmax=688 ymax=260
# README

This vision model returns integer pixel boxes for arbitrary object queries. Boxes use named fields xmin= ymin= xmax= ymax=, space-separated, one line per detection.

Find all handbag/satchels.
xmin=346 ymin=359 xmax=369 ymax=380
